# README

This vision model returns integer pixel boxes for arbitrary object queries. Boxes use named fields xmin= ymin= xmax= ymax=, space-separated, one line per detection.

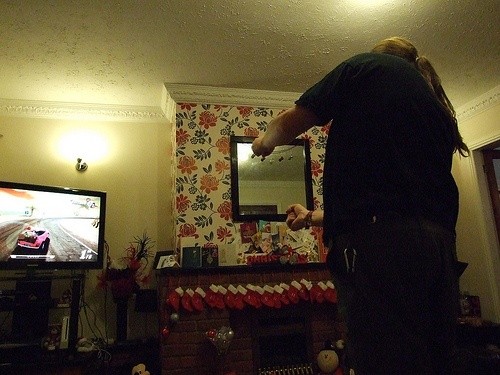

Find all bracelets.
xmin=304 ymin=210 xmax=314 ymax=230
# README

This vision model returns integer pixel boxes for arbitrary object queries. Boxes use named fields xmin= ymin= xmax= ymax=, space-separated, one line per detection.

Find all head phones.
xmin=77 ymin=338 xmax=94 ymax=352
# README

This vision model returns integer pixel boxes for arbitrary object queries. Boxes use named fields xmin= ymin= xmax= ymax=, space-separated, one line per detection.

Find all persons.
xmin=250 ymin=37 xmax=472 ymax=375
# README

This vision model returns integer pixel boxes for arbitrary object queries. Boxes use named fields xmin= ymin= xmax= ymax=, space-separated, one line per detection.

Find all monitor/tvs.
xmin=0 ymin=181 xmax=106 ymax=271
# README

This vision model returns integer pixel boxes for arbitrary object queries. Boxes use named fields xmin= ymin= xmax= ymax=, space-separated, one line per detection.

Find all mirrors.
xmin=230 ymin=134 xmax=314 ymax=222
